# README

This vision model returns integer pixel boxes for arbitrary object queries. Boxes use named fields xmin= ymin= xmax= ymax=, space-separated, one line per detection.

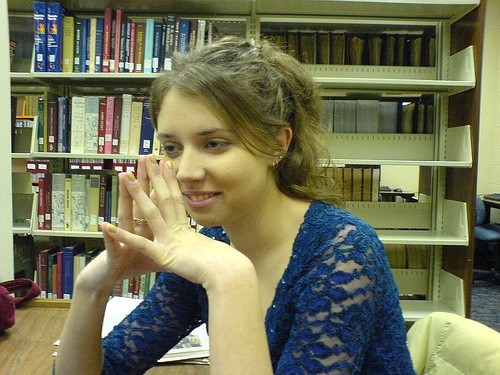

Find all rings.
xmin=131 ymin=216 xmax=148 ymax=226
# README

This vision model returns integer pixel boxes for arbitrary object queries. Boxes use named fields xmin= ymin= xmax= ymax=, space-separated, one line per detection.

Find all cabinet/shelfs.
xmin=7 ymin=0 xmax=487 ymax=331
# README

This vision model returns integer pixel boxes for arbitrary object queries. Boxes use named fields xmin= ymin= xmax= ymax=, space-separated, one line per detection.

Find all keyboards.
xmin=380 ymin=187 xmax=391 ymax=191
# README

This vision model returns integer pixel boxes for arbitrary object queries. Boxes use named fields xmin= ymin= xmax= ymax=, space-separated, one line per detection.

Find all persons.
xmin=53 ymin=35 xmax=417 ymax=375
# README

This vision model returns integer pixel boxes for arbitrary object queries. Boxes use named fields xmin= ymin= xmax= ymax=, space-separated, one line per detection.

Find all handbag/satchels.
xmin=0 ymin=278 xmax=41 ymax=330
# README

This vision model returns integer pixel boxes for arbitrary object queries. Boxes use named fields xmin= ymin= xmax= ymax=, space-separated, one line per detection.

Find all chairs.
xmin=472 ymin=193 xmax=500 ymax=284
xmin=405 ymin=312 xmax=500 ymax=375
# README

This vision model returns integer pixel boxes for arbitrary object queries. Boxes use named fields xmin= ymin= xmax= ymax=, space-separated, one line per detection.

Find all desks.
xmin=0 ymin=298 xmax=210 ymax=375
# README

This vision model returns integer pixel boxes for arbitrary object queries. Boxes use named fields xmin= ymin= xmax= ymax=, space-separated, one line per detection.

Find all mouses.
xmin=393 ymin=188 xmax=402 ymax=192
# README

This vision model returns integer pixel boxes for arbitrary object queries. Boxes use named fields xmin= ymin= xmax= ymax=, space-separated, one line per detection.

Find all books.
xmin=9 ymin=40 xmax=17 ymax=71
xmin=261 ymin=31 xmax=436 ymax=68
xmin=14 ymin=233 xmax=157 ymax=300
xmin=33 ymin=2 xmax=218 ymax=73
xmin=319 ymin=97 xmax=433 ymax=135
xmin=25 ymin=160 xmax=141 ymax=233
xmin=100 ymin=295 xmax=209 ymax=364
xmin=381 ymin=243 xmax=428 ymax=270
xmin=11 ymin=90 xmax=165 ymax=155
xmin=312 ymin=164 xmax=380 ymax=201
